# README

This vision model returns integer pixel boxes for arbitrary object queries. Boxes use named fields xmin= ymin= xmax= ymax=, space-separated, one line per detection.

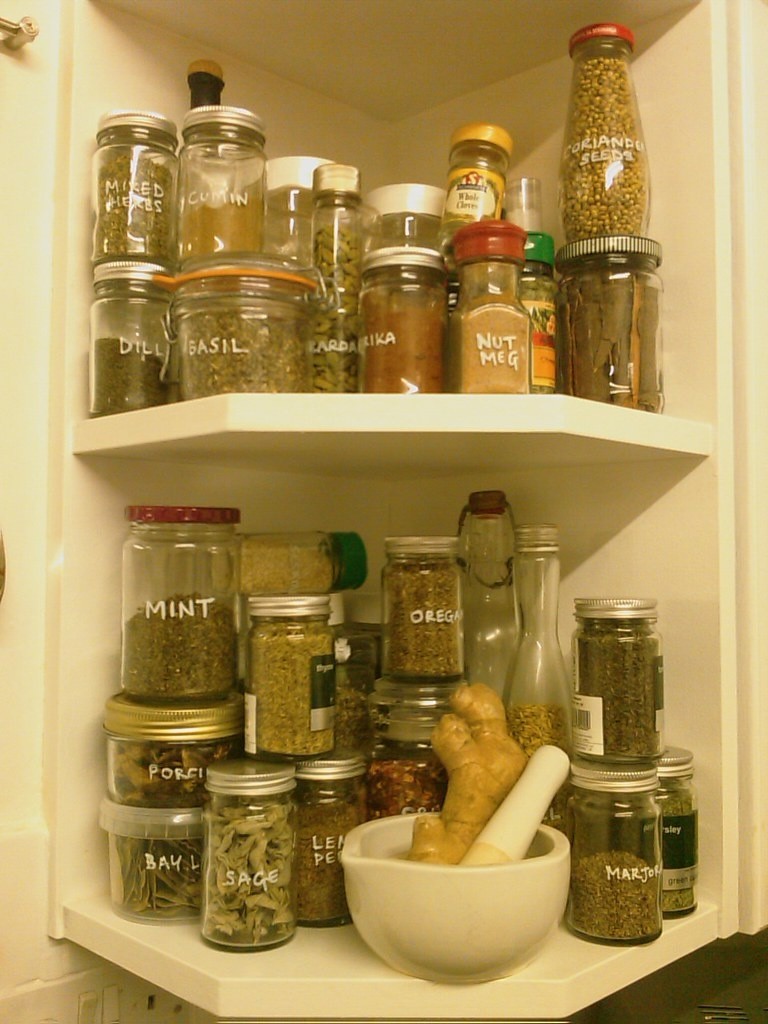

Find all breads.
xmin=87 ymin=104 xmax=664 ymax=419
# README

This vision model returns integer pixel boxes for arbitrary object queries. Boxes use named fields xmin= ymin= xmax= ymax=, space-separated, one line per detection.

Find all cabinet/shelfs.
xmin=39 ymin=0 xmax=749 ymax=1021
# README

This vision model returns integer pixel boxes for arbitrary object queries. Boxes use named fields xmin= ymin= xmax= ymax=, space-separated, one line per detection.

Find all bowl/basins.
xmin=341 ymin=808 xmax=572 ymax=985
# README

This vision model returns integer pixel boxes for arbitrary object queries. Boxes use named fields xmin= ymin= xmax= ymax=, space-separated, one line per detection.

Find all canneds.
xmin=97 ymin=505 xmax=699 ymax=952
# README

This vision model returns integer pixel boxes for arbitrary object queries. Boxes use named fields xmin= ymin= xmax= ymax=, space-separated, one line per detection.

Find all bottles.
xmin=89 ymin=23 xmax=666 ymax=414
xmin=100 ymin=489 xmax=701 ymax=948
